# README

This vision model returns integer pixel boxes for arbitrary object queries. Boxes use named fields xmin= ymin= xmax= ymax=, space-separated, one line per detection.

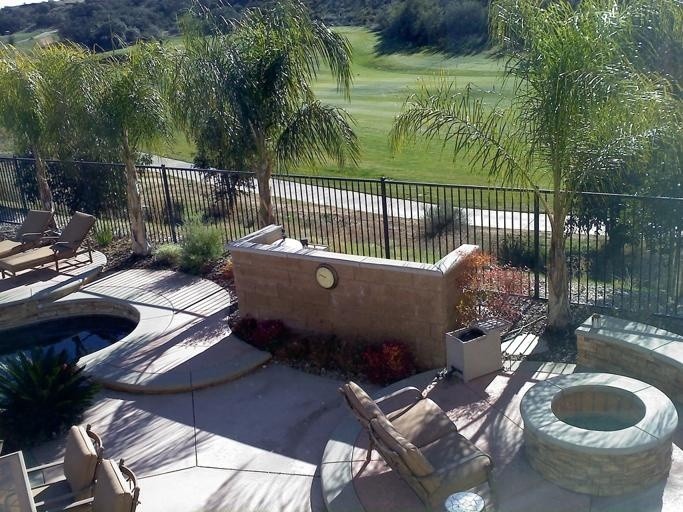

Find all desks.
xmin=0 ymin=450 xmax=38 ymax=511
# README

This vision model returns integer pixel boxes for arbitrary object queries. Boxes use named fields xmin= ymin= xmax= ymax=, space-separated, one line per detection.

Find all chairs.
xmin=0 ymin=209 xmax=60 ymax=259
xmin=55 ymin=460 xmax=142 ymax=512
xmin=0 ymin=210 xmax=96 ymax=284
xmin=27 ymin=424 xmax=102 ymax=505
xmin=363 ymin=415 xmax=501 ymax=512
xmin=340 ymin=380 xmax=457 ymax=479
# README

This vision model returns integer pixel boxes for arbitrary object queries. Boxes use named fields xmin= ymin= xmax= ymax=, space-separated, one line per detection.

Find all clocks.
xmin=315 ymin=263 xmax=339 ymax=289
